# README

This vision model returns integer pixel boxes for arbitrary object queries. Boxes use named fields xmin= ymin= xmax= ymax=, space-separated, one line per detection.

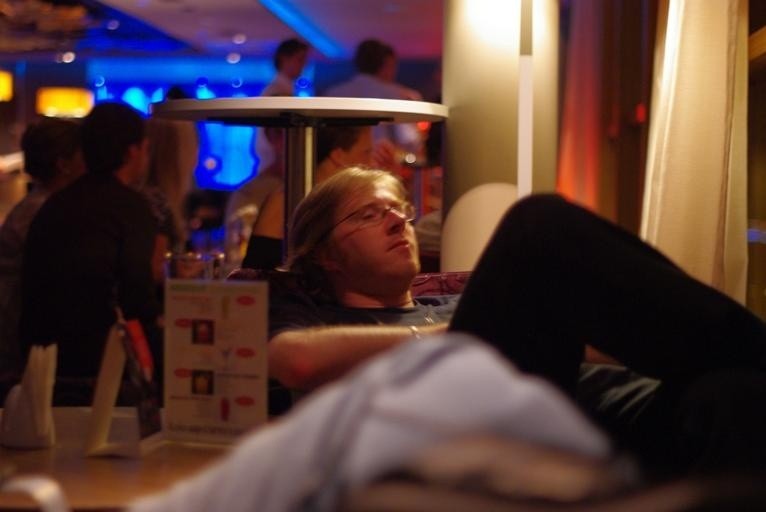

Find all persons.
xmin=220 ymin=35 xmax=433 ymax=275
xmin=1 ymin=85 xmax=197 ymax=429
xmin=267 ymin=166 xmax=766 ymax=512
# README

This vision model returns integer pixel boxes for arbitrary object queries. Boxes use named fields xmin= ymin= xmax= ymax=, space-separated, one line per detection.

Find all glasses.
xmin=328 ymin=203 xmax=416 ymax=228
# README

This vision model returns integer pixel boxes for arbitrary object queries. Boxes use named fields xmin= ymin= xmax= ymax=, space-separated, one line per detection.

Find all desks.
xmin=149 ymin=94 xmax=451 ymax=404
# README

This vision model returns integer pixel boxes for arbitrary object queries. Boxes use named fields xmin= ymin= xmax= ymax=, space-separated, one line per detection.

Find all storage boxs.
xmin=161 ymin=269 xmax=289 ymax=447
